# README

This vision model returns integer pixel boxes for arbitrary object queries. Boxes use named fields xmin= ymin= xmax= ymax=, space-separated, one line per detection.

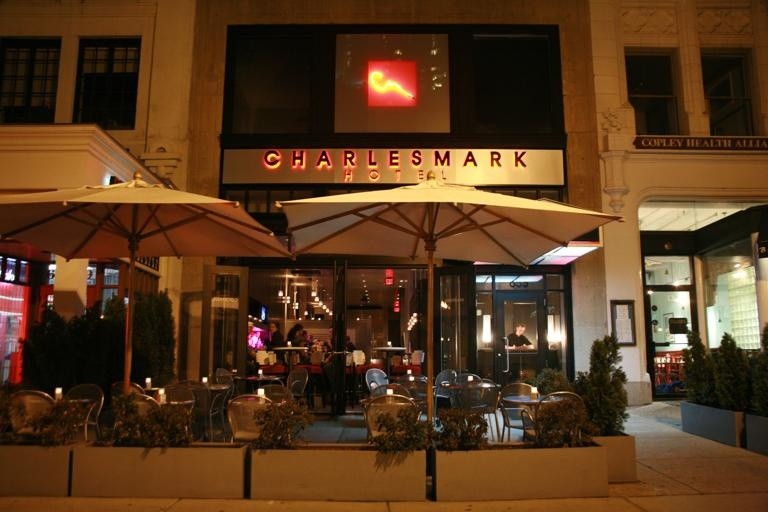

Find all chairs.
xmin=359 ymin=369 xmax=584 ymax=448
xmin=109 ymin=366 xmax=309 ymax=443
xmin=8 ymin=383 xmax=105 ymax=444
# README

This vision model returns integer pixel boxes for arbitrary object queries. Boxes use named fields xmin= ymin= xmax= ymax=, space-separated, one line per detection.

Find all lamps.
xmin=481 ymin=313 xmax=493 ymax=349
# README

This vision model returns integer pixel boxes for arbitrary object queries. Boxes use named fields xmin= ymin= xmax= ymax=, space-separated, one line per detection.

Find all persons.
xmin=507 ymin=321 xmax=535 ymax=350
xmin=245 ymin=318 xmax=357 ymax=407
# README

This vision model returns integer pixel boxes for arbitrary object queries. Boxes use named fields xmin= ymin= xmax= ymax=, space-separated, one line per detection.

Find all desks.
xmin=371 ymin=345 xmax=407 ymax=376
xmin=271 ymin=346 xmax=310 ymax=372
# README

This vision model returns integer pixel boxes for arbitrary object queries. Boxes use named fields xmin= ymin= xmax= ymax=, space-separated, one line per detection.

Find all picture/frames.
xmin=608 ymin=299 xmax=637 ymax=347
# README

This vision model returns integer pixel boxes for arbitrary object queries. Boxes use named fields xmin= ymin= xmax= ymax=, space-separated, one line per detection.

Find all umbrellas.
xmin=275 ymin=173 xmax=623 ymax=425
xmin=0 ymin=173 xmax=292 ymax=394
